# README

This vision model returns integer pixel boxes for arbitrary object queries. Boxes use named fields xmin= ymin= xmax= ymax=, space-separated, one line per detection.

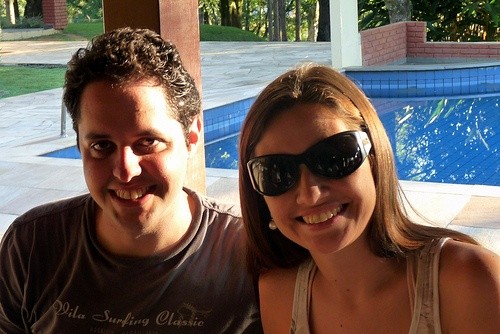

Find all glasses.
xmin=246 ymin=130 xmax=372 ymax=197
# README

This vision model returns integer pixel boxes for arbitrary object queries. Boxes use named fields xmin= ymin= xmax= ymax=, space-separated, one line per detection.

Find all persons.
xmin=0 ymin=26 xmax=263 ymax=334
xmin=237 ymin=68 xmax=500 ymax=333
